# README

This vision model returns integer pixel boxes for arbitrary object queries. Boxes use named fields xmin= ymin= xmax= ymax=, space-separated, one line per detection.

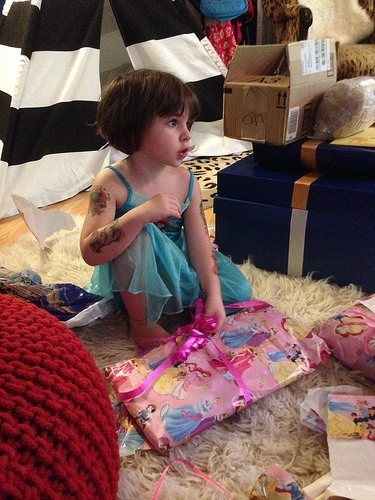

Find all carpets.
xmin=0 ymin=208 xmax=373 ymax=500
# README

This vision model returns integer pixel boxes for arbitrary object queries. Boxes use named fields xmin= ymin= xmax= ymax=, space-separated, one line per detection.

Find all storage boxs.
xmin=222 ymin=38 xmax=338 ymax=146
xmin=213 ymin=137 xmax=375 ymax=294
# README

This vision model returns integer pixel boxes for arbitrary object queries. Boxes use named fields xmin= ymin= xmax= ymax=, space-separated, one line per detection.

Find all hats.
xmin=0 ymin=294 xmax=119 ymax=500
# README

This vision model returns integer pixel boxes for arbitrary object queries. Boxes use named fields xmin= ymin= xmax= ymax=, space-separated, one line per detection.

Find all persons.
xmin=80 ymin=68 xmax=254 ymax=350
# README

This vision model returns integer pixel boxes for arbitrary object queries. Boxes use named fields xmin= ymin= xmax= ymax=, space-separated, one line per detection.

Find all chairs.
xmin=260 ymin=0 xmax=375 ymax=82
